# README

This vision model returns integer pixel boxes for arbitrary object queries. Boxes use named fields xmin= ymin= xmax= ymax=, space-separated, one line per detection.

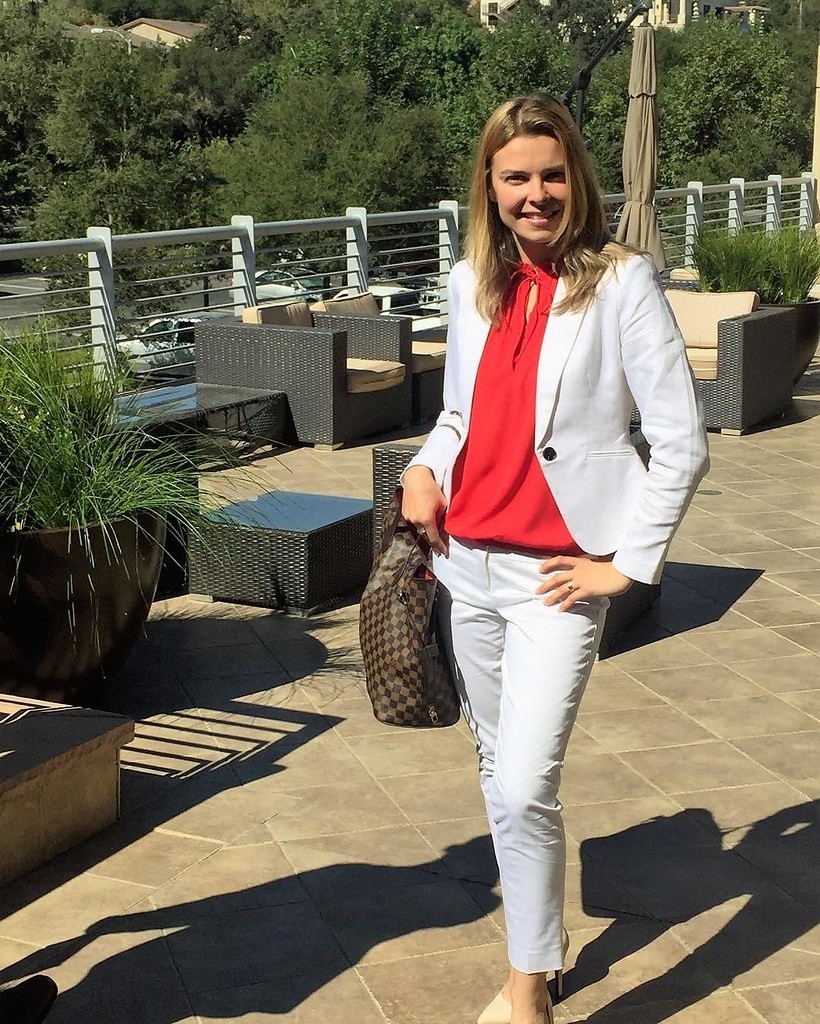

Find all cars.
xmin=230 ymin=267 xmax=340 ymax=306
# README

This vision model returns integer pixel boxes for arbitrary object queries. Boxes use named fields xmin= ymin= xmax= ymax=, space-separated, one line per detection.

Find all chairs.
xmin=626 ymin=286 xmax=795 ymax=437
xmin=309 ymin=291 xmax=450 ymax=427
xmin=191 ymin=300 xmax=414 ymax=450
xmin=372 ymin=428 xmax=661 ymax=665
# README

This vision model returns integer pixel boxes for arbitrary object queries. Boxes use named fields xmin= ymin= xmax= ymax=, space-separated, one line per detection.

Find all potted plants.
xmin=665 ymin=208 xmax=820 ymax=389
xmin=0 ymin=303 xmax=311 ymax=714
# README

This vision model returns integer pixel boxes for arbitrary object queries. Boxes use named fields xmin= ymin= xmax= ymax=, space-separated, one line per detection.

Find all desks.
xmin=110 ymin=381 xmax=282 ymax=463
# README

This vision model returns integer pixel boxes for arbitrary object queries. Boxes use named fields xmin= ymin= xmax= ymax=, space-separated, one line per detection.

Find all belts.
xmin=477 ymin=539 xmax=586 ymax=558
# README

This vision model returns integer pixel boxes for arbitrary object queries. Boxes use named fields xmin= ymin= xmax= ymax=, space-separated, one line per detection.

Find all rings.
xmin=417 ymin=530 xmax=425 ymax=536
xmin=567 ymin=582 xmax=574 ymax=592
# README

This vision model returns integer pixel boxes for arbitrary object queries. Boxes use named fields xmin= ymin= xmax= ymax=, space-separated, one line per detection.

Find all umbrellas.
xmin=615 ymin=12 xmax=668 ymax=274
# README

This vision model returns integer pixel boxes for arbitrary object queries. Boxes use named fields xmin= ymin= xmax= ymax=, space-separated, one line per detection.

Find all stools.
xmin=190 ymin=490 xmax=375 ymax=618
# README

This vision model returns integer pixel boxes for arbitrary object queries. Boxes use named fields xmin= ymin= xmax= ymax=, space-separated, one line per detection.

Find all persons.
xmin=399 ymin=94 xmax=711 ymax=1024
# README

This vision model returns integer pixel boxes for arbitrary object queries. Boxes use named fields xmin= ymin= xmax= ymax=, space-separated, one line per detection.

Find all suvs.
xmin=115 ymin=312 xmax=230 ymax=379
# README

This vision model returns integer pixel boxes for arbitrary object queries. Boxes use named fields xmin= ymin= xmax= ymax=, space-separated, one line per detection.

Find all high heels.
xmin=545 ymin=988 xmax=553 ymax=1024
xmin=476 ymin=928 xmax=570 ymax=1024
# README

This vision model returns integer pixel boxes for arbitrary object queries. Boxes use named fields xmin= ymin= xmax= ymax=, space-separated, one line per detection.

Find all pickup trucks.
xmin=328 ymin=286 xmax=440 ymax=327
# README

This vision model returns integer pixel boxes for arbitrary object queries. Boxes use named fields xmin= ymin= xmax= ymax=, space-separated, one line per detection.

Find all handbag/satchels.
xmin=359 ymin=486 xmax=461 ymax=728
